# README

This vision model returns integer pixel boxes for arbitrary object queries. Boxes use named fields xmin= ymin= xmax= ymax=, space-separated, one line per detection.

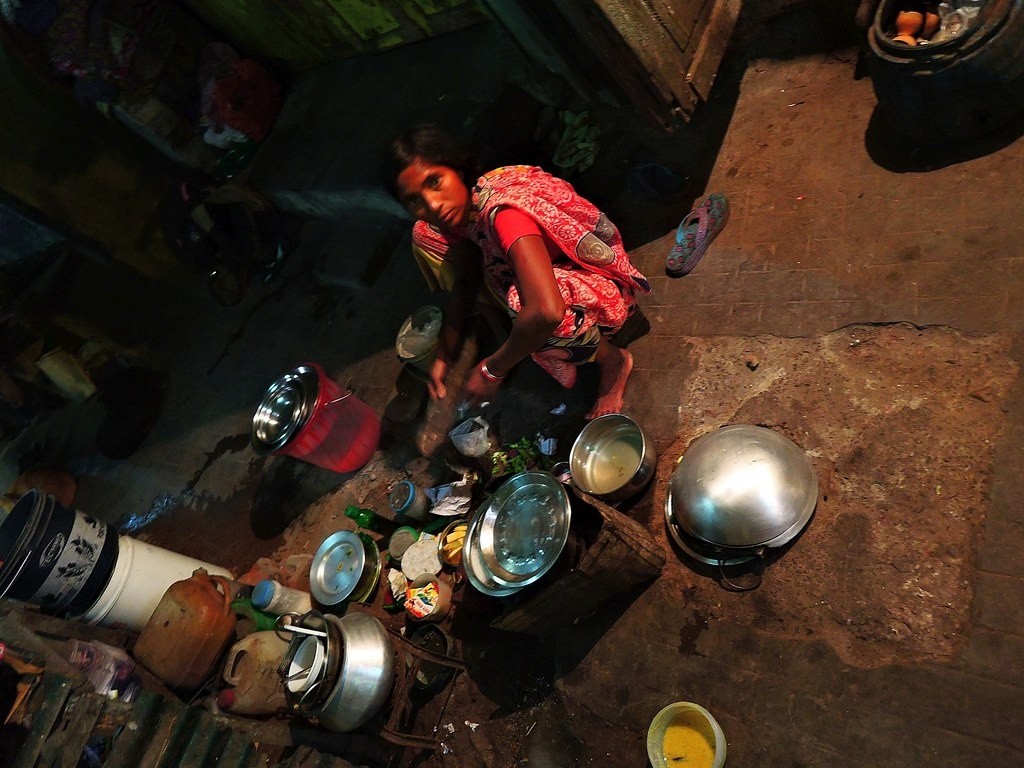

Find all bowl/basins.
xmin=646 ymin=701 xmax=727 ymax=768
xmin=250 ymin=375 xmax=308 ymax=456
xmin=569 ymin=413 xmax=657 ymax=500
xmin=406 ymin=573 xmax=453 ymax=623
xmin=287 ymin=635 xmax=324 ymax=693
xmin=437 ymin=519 xmax=470 ymax=567
xmin=462 ymin=497 xmax=525 ymax=597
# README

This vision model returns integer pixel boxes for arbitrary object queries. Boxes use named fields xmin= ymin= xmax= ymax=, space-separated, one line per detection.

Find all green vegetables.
xmin=486 ymin=387 xmax=556 ymax=475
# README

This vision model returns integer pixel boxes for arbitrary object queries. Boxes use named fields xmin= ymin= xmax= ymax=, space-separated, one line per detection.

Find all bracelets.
xmin=479 ymin=356 xmax=505 ymax=382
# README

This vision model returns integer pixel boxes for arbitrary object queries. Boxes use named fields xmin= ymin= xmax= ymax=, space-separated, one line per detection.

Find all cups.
xmin=389 ymin=480 xmax=429 ymax=522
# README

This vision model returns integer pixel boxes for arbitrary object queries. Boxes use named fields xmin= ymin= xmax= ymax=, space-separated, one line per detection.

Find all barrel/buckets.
xmin=217 ymin=631 xmax=293 ymax=713
xmin=82 ymin=534 xmax=234 ymax=634
xmin=0 ymin=488 xmax=118 ymax=620
xmin=272 ymin=362 xmax=381 ymax=472
xmin=396 ymin=305 xmax=443 ymax=374
xmin=132 ymin=568 xmax=236 ymax=700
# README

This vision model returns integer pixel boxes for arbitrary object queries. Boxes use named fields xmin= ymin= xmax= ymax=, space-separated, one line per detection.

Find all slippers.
xmin=665 ymin=192 xmax=729 ymax=276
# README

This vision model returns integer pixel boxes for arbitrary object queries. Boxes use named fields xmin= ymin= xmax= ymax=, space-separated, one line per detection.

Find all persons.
xmin=377 ymin=125 xmax=650 ymax=420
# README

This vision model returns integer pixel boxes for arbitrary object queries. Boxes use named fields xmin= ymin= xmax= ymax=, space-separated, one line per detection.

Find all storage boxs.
xmin=490 ymin=479 xmax=668 ymax=640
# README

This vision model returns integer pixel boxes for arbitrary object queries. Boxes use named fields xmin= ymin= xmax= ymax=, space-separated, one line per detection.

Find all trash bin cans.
xmin=853 ymin=0 xmax=1023 ymax=138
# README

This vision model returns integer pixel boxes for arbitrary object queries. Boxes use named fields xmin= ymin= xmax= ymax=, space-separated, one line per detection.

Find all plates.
xmin=310 ymin=531 xmax=365 ymax=606
xmin=346 ymin=531 xmax=381 ymax=602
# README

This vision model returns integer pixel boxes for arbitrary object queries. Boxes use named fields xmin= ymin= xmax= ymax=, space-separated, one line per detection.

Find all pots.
xmin=283 ymin=611 xmax=344 ymax=712
xmin=672 ymin=425 xmax=818 ymax=548
xmin=274 ymin=610 xmax=396 ymax=732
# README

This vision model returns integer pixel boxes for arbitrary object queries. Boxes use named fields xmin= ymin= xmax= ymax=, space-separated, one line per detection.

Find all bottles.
xmin=252 ymin=580 xmax=312 ymax=616
xmin=345 ymin=505 xmax=394 ymax=535
